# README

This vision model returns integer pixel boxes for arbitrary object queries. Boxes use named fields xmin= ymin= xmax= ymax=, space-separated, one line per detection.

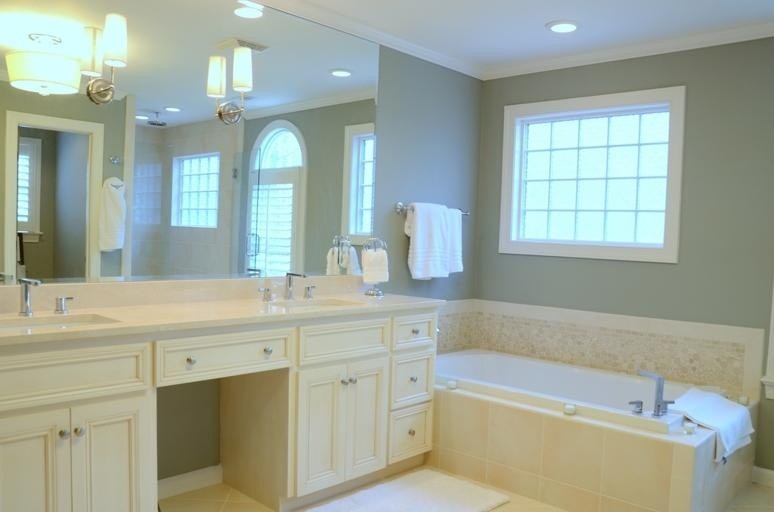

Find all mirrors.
xmin=0 ymin=1 xmax=381 ymax=286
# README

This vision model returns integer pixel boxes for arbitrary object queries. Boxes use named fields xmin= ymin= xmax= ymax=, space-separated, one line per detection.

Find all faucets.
xmin=637 ymin=369 xmax=667 ymax=417
xmin=283 ymin=273 xmax=307 ymax=300
xmin=17 ymin=276 xmax=41 ymax=316
xmin=0 ymin=272 xmax=14 ymax=286
xmin=246 ymin=267 xmax=264 ymax=279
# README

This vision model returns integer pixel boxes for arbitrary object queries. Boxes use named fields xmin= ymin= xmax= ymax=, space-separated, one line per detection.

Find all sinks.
xmin=268 ymin=297 xmax=365 ymax=310
xmin=0 ymin=313 xmax=123 ymax=334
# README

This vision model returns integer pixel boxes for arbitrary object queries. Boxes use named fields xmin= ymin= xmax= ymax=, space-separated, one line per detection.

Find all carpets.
xmin=305 ymin=466 xmax=514 ymax=511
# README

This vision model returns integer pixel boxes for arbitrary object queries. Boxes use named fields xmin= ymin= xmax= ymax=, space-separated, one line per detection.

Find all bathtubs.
xmin=426 ymin=346 xmax=724 ymax=428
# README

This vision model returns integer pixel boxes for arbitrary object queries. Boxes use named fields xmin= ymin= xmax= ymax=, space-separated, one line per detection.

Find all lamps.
xmin=208 ymin=46 xmax=254 ymax=124
xmin=4 ymin=3 xmax=129 ymax=115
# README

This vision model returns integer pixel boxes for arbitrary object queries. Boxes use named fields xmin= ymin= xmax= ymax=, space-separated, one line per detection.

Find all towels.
xmin=672 ymin=385 xmax=755 ymax=461
xmin=327 ymin=244 xmax=358 ymax=277
xmin=98 ymin=174 xmax=125 ymax=254
xmin=361 ymin=245 xmax=387 ymax=284
xmin=404 ymin=200 xmax=467 ymax=283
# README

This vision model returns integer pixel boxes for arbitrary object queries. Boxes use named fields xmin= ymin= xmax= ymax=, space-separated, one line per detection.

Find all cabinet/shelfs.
xmin=0 ymin=339 xmax=161 ymax=512
xmin=389 ymin=311 xmax=442 ymax=477
xmin=217 ymin=315 xmax=387 ymax=510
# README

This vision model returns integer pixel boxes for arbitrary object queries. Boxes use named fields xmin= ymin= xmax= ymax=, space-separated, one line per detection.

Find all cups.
xmin=332 ymin=234 xmax=350 ymax=267
xmin=363 ymin=238 xmax=384 ymax=252
